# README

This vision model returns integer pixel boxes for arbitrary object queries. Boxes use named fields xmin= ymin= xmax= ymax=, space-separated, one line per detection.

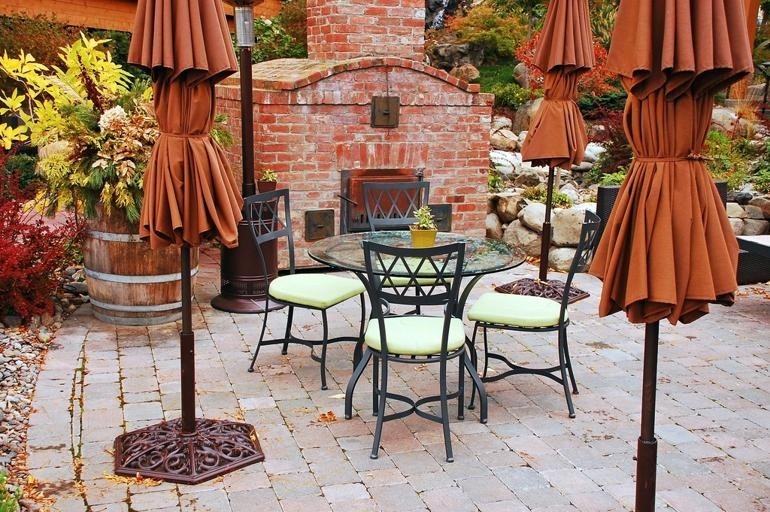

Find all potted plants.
xmin=256 ymin=165 xmax=278 ymax=194
xmin=407 ymin=204 xmax=438 ymax=251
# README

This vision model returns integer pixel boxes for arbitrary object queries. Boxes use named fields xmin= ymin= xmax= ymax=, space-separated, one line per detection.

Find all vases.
xmin=76 ymin=192 xmax=202 ymax=327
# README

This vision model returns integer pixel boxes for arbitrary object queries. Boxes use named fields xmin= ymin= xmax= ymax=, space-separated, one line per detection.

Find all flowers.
xmin=0 ymin=24 xmax=234 ymax=238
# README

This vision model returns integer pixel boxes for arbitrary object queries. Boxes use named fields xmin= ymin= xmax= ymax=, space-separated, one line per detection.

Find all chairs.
xmin=238 ymin=186 xmax=371 ymax=393
xmin=461 ymin=207 xmax=604 ymax=424
xmin=354 ymin=181 xmax=431 ymax=378
xmin=340 ymin=236 xmax=493 ymax=463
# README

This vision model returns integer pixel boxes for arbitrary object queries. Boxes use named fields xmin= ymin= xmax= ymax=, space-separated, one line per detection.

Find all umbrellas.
xmin=521 ymin=0 xmax=596 ymax=282
xmin=587 ymin=0 xmax=754 ymax=512
xmin=126 ymin=0 xmax=246 ymax=433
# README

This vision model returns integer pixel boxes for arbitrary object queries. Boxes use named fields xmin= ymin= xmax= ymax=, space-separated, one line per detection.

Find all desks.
xmin=306 ymin=229 xmax=530 ymax=430
